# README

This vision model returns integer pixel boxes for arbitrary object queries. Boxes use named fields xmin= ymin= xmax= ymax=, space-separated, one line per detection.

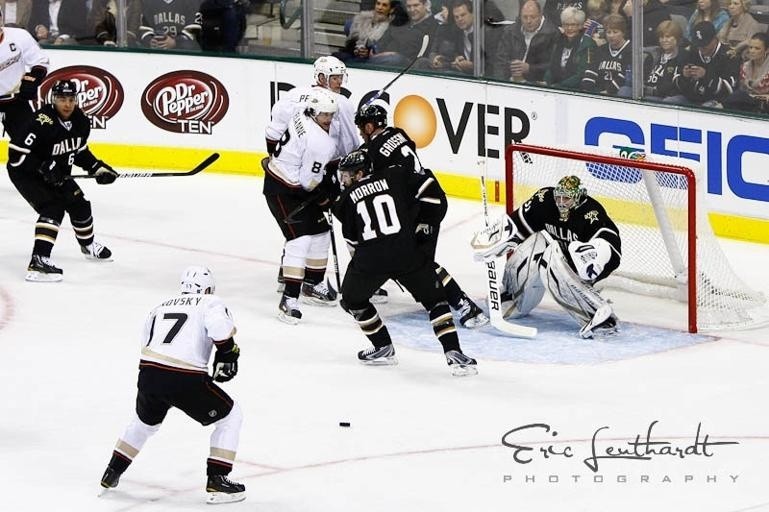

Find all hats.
xmin=692 ymin=21 xmax=715 ymax=47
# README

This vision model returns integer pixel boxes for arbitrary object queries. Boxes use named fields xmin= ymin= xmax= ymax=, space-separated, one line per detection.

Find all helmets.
xmin=340 ymin=150 xmax=368 ymax=171
xmin=180 ymin=266 xmax=215 ymax=293
xmin=355 ymin=105 xmax=387 ymax=124
xmin=314 ymin=56 xmax=346 ymax=81
xmin=553 ymin=176 xmax=587 ymax=214
xmin=52 ymin=80 xmax=76 ymax=95
xmin=306 ymin=90 xmax=338 ymax=112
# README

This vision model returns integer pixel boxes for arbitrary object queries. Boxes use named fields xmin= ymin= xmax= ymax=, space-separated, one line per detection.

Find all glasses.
xmin=561 ymin=24 xmax=577 ymax=27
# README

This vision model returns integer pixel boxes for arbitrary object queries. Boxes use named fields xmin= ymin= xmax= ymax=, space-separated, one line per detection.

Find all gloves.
xmin=570 ymin=241 xmax=607 ymax=282
xmin=21 ymin=66 xmax=47 ymax=99
xmin=469 ymin=222 xmax=508 ymax=261
xmin=38 ymin=160 xmax=67 ymax=194
xmin=212 ymin=345 xmax=238 ymax=382
xmin=89 ymin=160 xmax=119 ymax=184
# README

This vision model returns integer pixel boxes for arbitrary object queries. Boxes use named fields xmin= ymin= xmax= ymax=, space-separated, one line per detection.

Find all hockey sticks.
xmin=477 ymin=158 xmax=538 ymax=339
xmin=326 ymin=276 xmax=342 ymax=300
xmin=65 ymin=153 xmax=219 ymax=178
xmin=351 ymin=35 xmax=429 ymax=115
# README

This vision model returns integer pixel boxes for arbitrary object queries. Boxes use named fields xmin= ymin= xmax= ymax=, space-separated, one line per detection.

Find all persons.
xmin=0 ymin=0 xmax=247 ymax=53
xmin=497 ymin=172 xmax=623 ymax=335
xmin=0 ymin=8 xmax=50 ymax=139
xmin=354 ymin=104 xmax=490 ymax=328
xmin=101 ymin=263 xmax=246 ymax=495
xmin=262 ymin=91 xmax=338 ymax=327
xmin=331 ymin=150 xmax=481 ymax=375
xmin=345 ymin=1 xmax=767 ymax=116
xmin=266 ymin=55 xmax=391 ymax=303
xmin=6 ymin=78 xmax=121 ymax=274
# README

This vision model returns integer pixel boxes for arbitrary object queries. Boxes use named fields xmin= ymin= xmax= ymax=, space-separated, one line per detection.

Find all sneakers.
xmin=81 ymin=242 xmax=111 ymax=258
xmin=101 ymin=468 xmax=119 ymax=488
xmin=28 ymin=255 xmax=62 ymax=273
xmin=460 ymin=292 xmax=482 ymax=325
xmin=358 ymin=345 xmax=394 ymax=360
xmin=446 ymin=350 xmax=477 ymax=366
xmin=374 ymin=288 xmax=387 ymax=295
xmin=302 ymin=282 xmax=336 ymax=301
xmin=279 ymin=294 xmax=301 ymax=319
xmin=206 ymin=475 xmax=245 ymax=493
xmin=592 ymin=317 xmax=616 ymax=332
